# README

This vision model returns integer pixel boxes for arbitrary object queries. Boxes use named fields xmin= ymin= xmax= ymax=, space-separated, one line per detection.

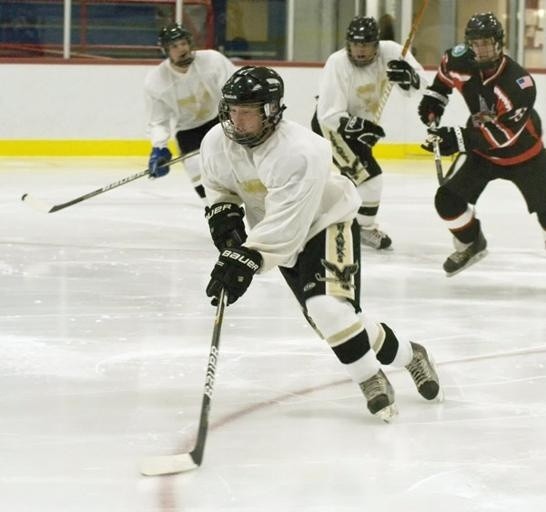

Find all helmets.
xmin=464 ymin=11 xmax=504 ymax=70
xmin=155 ymin=24 xmax=196 ymax=68
xmin=346 ymin=15 xmax=382 ymax=69
xmin=217 ymin=64 xmax=288 ymax=149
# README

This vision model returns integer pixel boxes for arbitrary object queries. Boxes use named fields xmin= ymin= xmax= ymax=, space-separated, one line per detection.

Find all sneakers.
xmin=358 ymin=369 xmax=396 ymax=415
xmin=360 ymin=225 xmax=393 ymax=250
xmin=404 ymin=340 xmax=440 ymax=401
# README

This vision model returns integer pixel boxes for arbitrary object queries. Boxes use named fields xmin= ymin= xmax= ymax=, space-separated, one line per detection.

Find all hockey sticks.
xmin=428 ymin=113 xmax=468 ymax=186
xmin=21 ymin=149 xmax=200 ymax=215
xmin=142 ymin=230 xmax=240 ymax=476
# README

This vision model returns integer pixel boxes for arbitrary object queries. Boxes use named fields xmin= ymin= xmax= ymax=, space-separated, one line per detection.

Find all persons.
xmin=312 ymin=16 xmax=430 ymax=250
xmin=198 ymin=65 xmax=445 ymax=422
xmin=420 ymin=14 xmax=546 ymax=276
xmin=142 ymin=23 xmax=240 ymax=213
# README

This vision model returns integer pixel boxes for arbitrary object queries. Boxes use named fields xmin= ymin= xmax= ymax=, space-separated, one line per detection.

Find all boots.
xmin=442 ymin=220 xmax=488 ymax=273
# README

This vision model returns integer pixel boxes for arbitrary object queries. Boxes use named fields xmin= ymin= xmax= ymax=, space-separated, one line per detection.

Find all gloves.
xmin=419 ymin=124 xmax=471 ymax=157
xmin=206 ymin=245 xmax=263 ymax=310
xmin=203 ymin=200 xmax=248 ymax=253
xmin=387 ymin=58 xmax=422 ymax=94
xmin=147 ymin=145 xmax=173 ymax=179
xmin=338 ymin=116 xmax=385 ymax=157
xmin=418 ymin=84 xmax=450 ymax=129
